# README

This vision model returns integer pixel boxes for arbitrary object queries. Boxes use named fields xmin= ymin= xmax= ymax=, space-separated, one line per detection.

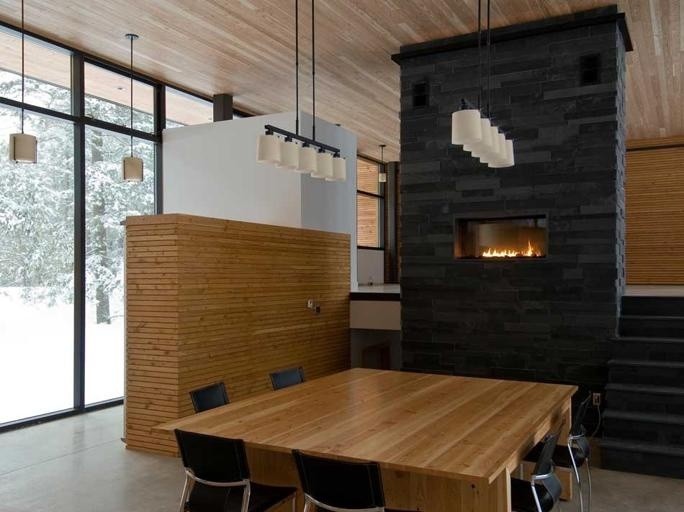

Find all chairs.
xmin=511 ymin=415 xmax=566 ymax=512
xmin=269 ymin=366 xmax=306 ymax=391
xmin=174 ymin=429 xmax=297 ymax=512
xmin=189 ymin=382 xmax=229 ymax=414
xmin=522 ymin=391 xmax=601 ymax=512
xmin=292 ymin=450 xmax=385 ymax=511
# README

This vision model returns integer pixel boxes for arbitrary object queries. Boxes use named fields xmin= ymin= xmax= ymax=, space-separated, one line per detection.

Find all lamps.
xmin=10 ymin=0 xmax=37 ymax=164
xmin=479 ymin=134 xmax=507 ymax=163
xmin=471 ymin=127 xmax=498 ymax=158
xmin=463 ymin=118 xmax=491 ymax=152
xmin=488 ymin=139 xmax=515 ymax=168
xmin=299 ymin=141 xmax=316 ymax=175
xmin=379 ymin=144 xmax=387 ymax=183
xmin=281 ymin=136 xmax=299 ymax=171
xmin=256 ymin=130 xmax=281 ymax=165
xmin=123 ymin=34 xmax=143 ymax=182
xmin=311 ymin=147 xmax=333 ymax=179
xmin=451 ymin=109 xmax=481 ymax=145
xmin=326 ymin=153 xmax=346 ymax=183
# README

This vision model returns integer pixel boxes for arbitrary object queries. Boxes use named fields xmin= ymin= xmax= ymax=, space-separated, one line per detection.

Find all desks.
xmin=152 ymin=367 xmax=578 ymax=512
